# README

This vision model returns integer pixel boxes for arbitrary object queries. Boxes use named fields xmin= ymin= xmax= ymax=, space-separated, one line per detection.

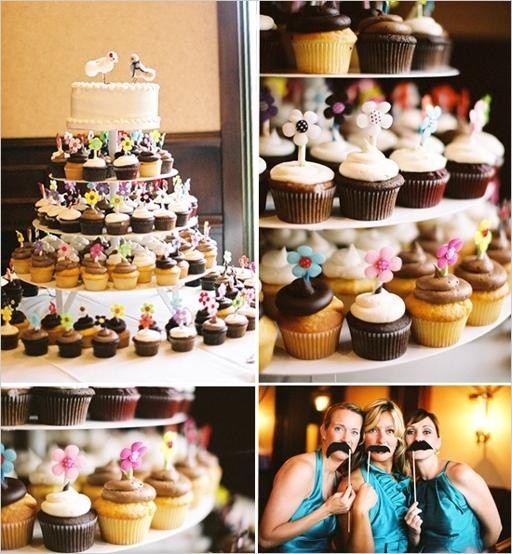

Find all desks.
xmin=0 ymin=266 xmax=255 ymax=383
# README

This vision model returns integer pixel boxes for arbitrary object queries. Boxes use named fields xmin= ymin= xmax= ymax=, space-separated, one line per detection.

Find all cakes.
xmin=1 ymin=1 xmax=511 ymax=361
xmin=1 ymin=387 xmax=223 ymax=554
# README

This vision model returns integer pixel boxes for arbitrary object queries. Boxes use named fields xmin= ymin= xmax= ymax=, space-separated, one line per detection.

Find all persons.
xmin=259 ymin=402 xmax=365 ymax=554
xmin=402 ymin=408 xmax=503 ymax=554
xmin=336 ymin=399 xmax=413 ymax=553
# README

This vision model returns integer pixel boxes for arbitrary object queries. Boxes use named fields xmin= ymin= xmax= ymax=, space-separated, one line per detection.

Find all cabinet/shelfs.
xmin=6 ymin=125 xmax=218 ymax=331
xmin=0 ymin=413 xmax=216 ymax=554
xmin=257 ymin=67 xmax=512 ymax=376
